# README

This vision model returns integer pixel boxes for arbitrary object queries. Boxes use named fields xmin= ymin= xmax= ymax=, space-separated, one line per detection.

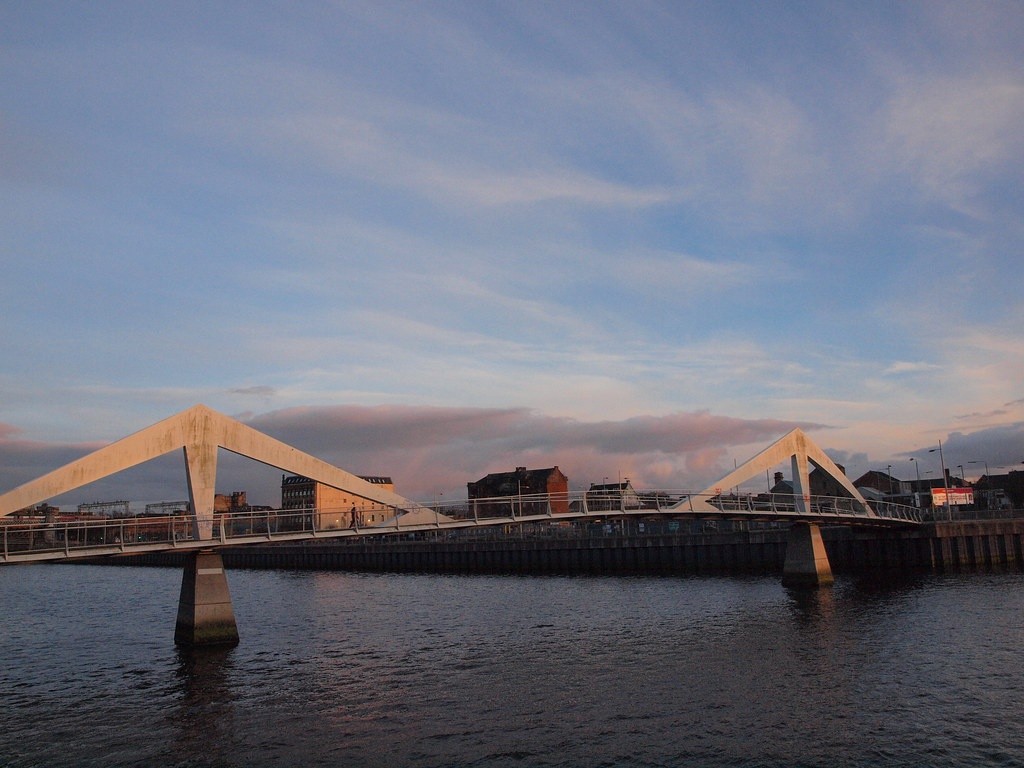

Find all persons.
xmin=348 ymin=506 xmax=361 ymax=527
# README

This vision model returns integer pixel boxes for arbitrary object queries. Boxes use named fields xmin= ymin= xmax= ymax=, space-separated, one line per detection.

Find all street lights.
xmin=928 ymin=439 xmax=952 ymax=522
xmin=968 ymin=460 xmax=993 ymax=511
xmin=433 ymin=488 xmax=443 ymax=523
xmin=957 ymin=464 xmax=970 ymax=511
xmin=603 ymin=475 xmax=610 ymax=526
xmin=909 ymin=458 xmax=920 ymax=493
xmin=517 ymin=479 xmax=530 ymax=534
xmin=618 ymin=469 xmax=630 ymax=536
xmin=886 ymin=464 xmax=894 ymax=518
xmin=917 ymin=468 xmax=934 ymax=493
